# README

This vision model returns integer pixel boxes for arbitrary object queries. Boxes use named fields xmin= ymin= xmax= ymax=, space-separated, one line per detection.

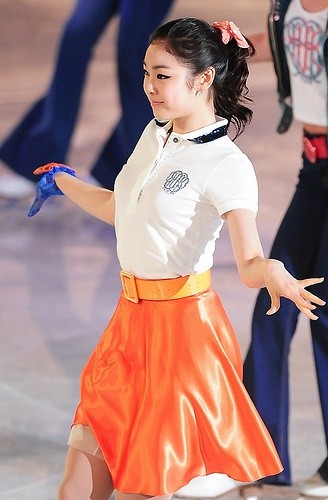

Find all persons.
xmin=0 ymin=0 xmax=176 ymax=209
xmin=28 ymin=17 xmax=326 ymax=500
xmin=232 ymin=0 xmax=328 ymax=497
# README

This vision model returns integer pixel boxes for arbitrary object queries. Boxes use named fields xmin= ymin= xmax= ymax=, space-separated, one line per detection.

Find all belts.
xmin=119 ymin=268 xmax=212 ymax=305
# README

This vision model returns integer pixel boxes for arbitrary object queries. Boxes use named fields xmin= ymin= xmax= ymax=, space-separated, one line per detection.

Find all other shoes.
xmin=0 ymin=174 xmax=35 ymax=204
xmin=300 ymin=473 xmax=328 ymax=496
